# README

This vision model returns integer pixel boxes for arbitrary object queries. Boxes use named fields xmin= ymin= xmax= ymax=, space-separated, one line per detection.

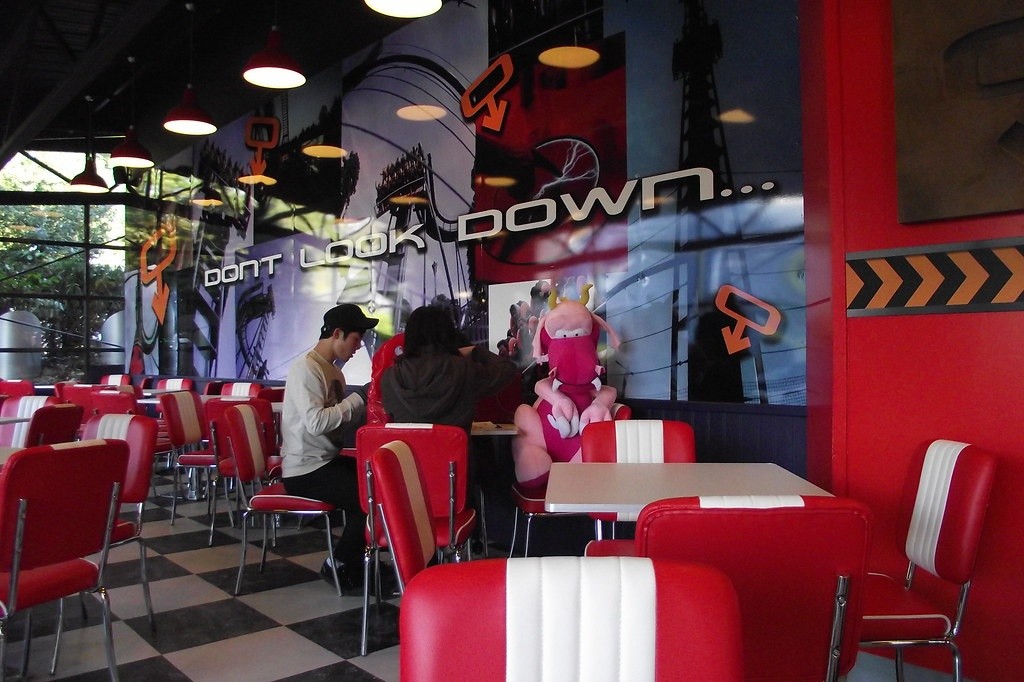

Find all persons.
xmin=497 ymin=276 xmax=587 ymax=360
xmin=380 ymin=305 xmax=516 ymax=510
xmin=280 ymin=303 xmax=379 ymax=596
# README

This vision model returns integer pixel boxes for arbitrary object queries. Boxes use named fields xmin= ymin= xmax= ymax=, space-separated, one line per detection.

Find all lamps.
xmin=190 ymin=178 xmax=222 ymax=207
xmin=110 ymin=70 xmax=155 ymax=168
xmin=160 ymin=0 xmax=218 ymax=136
xmin=70 ymin=103 xmax=110 ymax=194
xmin=239 ymin=0 xmax=308 ymax=90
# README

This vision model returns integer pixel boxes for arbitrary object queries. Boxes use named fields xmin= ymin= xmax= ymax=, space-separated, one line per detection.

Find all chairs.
xmin=140 ymin=376 xmax=154 ymax=389
xmin=857 ymin=439 xmax=1001 ymax=682
xmin=202 ymin=381 xmax=262 ymax=398
xmin=100 ymin=375 xmax=131 ymax=386
xmin=635 ymin=496 xmax=873 ymax=682
xmin=508 ymin=404 xmax=632 ymax=557
xmin=227 ymin=405 xmax=344 ymax=597
xmin=581 ymin=418 xmax=698 ymax=541
xmin=160 ymin=391 xmax=247 ymax=527
xmin=398 ymin=556 xmax=745 ymax=682
xmin=156 ymin=378 xmax=192 ymax=418
xmin=355 ymin=423 xmax=476 ymax=656
xmin=206 ymin=399 xmax=278 ymax=548
xmin=0 ymin=378 xmax=173 ymax=682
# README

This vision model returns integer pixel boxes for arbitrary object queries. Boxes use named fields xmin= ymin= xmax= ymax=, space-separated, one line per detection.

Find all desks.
xmin=0 ymin=416 xmax=32 ymax=425
xmin=34 ymin=384 xmax=93 ymax=390
xmin=544 ymin=461 xmax=838 ymax=541
xmin=0 ymin=447 xmax=27 ymax=466
xmin=222 ymin=398 xmax=283 ymax=413
xmin=471 ymin=422 xmax=519 ymax=435
xmin=136 ymin=394 xmax=222 ymax=404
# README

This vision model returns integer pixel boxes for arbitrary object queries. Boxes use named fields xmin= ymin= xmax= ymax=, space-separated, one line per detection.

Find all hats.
xmin=324 ymin=304 xmax=379 ymax=329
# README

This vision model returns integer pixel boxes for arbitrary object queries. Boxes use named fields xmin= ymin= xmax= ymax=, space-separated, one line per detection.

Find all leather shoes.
xmin=356 ymin=556 xmax=393 ymax=579
xmin=321 ymin=559 xmax=364 ymax=595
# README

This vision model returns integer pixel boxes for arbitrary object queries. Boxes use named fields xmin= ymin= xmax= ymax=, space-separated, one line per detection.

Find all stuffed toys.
xmin=515 ymin=282 xmax=621 ymax=486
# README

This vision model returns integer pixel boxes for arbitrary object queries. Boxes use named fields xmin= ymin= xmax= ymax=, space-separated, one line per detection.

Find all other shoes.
xmin=471 ymin=540 xmax=484 ymax=554
